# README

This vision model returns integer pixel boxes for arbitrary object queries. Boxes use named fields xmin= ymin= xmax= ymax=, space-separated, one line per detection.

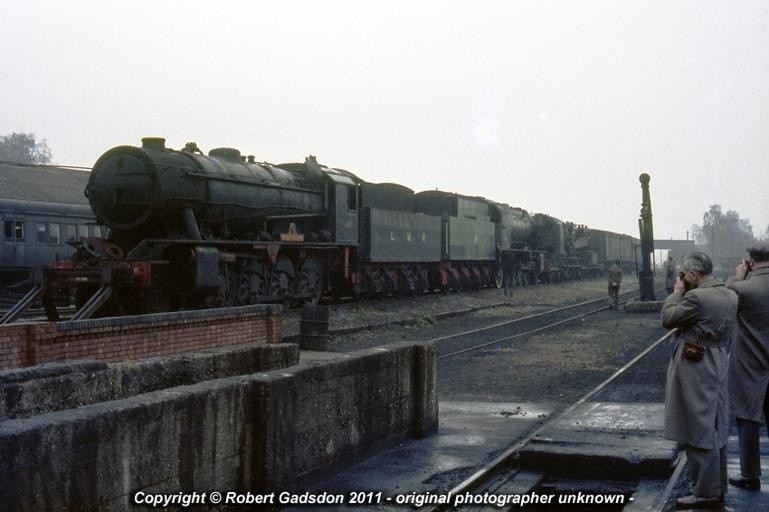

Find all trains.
xmin=39 ymin=137 xmax=642 ymax=316
xmin=0 ymin=197 xmax=110 ymax=288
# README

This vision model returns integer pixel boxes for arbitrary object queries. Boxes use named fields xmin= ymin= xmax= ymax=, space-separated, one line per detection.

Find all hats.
xmin=746 ymin=241 xmax=768 ymax=255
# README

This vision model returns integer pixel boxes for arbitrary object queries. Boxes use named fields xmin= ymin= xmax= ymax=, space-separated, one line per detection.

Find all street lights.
xmin=638 ymin=174 xmax=654 ymax=302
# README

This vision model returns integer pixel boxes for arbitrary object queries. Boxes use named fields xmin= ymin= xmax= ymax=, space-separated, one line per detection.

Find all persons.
xmin=725 ymin=241 xmax=769 ymax=492
xmin=666 ymin=257 xmax=676 ymax=295
xmin=661 ymin=252 xmax=739 ymax=508
xmin=607 ymin=260 xmax=623 ymax=311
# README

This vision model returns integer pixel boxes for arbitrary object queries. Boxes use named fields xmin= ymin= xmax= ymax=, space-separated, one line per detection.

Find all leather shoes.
xmin=676 ymin=495 xmax=719 ymax=507
xmin=729 ymin=476 xmax=761 ymax=492
